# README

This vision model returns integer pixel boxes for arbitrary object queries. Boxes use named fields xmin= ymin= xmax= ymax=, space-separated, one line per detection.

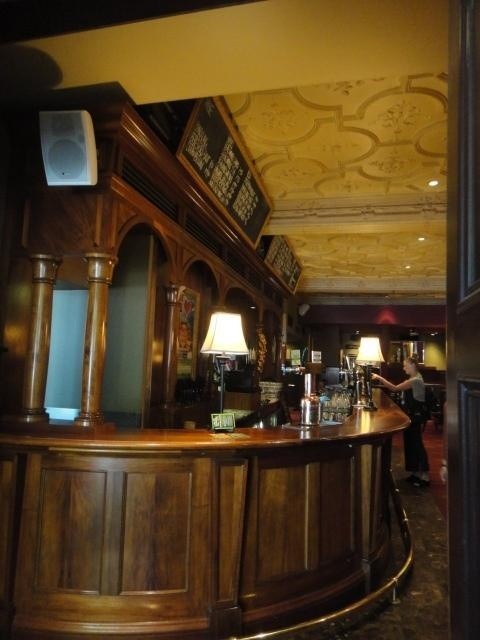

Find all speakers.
xmin=39 ymin=110 xmax=98 ymax=186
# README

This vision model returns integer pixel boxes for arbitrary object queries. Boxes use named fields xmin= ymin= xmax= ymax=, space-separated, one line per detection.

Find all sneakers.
xmin=398 ymin=475 xmax=431 ymax=486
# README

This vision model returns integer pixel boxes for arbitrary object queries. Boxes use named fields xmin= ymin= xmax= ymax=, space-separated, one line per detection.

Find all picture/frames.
xmin=178 ymin=287 xmax=201 ymax=382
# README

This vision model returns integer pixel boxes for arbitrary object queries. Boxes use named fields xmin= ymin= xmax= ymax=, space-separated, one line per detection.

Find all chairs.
xmin=278 ymin=390 xmax=299 ymax=424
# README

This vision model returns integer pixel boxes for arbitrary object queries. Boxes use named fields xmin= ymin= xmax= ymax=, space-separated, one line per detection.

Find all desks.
xmin=423 ymin=382 xmax=447 ymax=433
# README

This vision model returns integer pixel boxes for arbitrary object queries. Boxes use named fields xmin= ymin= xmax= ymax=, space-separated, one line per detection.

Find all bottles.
xmin=301 ymin=392 xmax=321 ymax=426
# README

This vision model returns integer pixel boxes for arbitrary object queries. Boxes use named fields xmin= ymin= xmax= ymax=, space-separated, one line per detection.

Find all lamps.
xmin=354 ymin=335 xmax=386 ymax=411
xmin=199 ymin=311 xmax=250 ymax=434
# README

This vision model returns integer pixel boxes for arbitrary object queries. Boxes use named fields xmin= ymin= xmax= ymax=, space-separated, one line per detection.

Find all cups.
xmin=289 ymin=407 xmax=301 ymax=426
xmin=321 ymin=393 xmax=352 ymax=422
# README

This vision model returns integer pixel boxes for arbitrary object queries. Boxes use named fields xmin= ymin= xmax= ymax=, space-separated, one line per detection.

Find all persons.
xmin=370 ymin=353 xmax=433 ymax=487
xmin=177 ymin=319 xmax=193 ymax=353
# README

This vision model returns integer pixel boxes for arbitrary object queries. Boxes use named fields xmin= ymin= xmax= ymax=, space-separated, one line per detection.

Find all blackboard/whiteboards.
xmin=178 ymin=97 xmax=273 ymax=247
xmin=265 ymin=236 xmax=304 ymax=294
